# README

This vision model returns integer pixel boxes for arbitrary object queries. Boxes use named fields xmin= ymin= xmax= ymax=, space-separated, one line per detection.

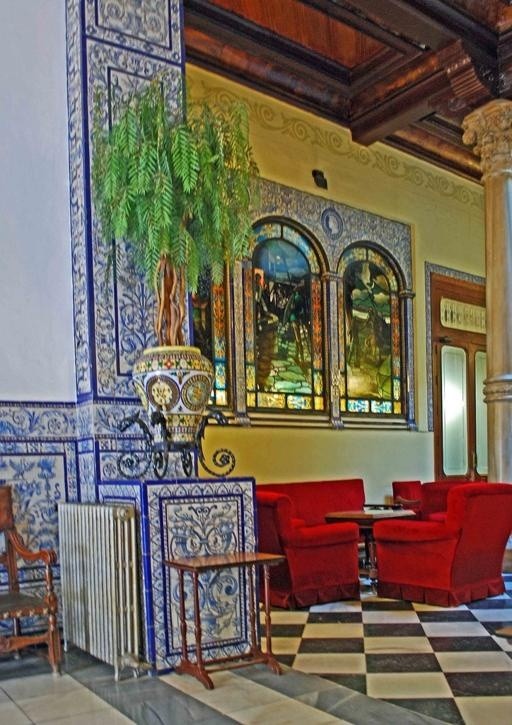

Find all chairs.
xmin=392 ymin=481 xmax=422 ymax=515
xmin=254 ymin=491 xmax=360 ymax=611
xmin=0 ymin=484 xmax=63 ymax=677
xmin=416 ymin=480 xmax=472 ymax=522
xmin=372 ymin=483 xmax=512 ymax=608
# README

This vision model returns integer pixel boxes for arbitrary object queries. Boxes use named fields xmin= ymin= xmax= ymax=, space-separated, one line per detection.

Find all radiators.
xmin=55 ymin=498 xmax=155 ymax=683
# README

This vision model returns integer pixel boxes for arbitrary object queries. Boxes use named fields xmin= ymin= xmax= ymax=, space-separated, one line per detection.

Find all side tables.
xmin=363 ymin=504 xmax=402 ymax=566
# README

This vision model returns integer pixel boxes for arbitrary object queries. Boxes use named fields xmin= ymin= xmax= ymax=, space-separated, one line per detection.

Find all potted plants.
xmin=85 ymin=69 xmax=264 ymax=447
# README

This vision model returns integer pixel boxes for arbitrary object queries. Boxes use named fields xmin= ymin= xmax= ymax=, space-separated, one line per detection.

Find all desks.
xmin=160 ymin=551 xmax=285 ymax=691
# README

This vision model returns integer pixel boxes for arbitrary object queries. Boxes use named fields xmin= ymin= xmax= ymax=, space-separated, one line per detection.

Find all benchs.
xmin=255 ymin=478 xmax=366 ymax=546
